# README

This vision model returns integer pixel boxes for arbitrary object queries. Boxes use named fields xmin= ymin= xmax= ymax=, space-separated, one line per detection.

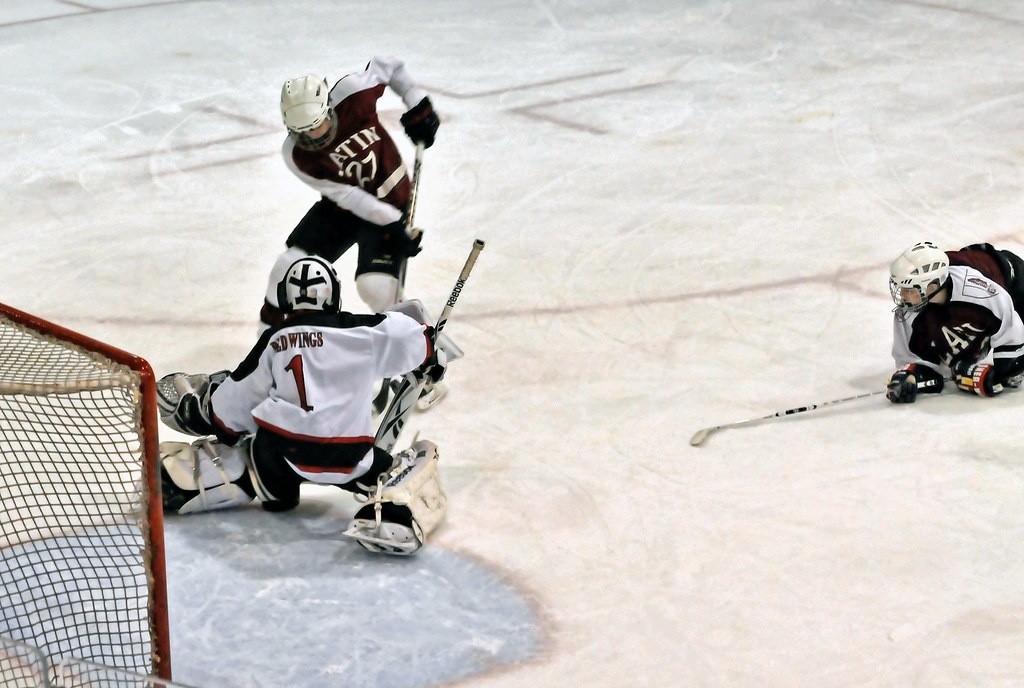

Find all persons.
xmin=156 ymin=255 xmax=446 ymax=558
xmin=254 ymin=55 xmax=447 ymax=409
xmin=885 ymin=241 xmax=1024 ymax=403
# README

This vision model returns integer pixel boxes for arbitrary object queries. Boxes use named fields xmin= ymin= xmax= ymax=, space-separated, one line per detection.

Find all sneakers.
xmin=417 ymin=381 xmax=447 ymax=410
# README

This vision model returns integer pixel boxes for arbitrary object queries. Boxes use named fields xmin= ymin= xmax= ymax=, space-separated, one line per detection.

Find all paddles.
xmin=393 ymin=142 xmax=423 ymax=303
xmin=371 ymin=238 xmax=485 ymax=452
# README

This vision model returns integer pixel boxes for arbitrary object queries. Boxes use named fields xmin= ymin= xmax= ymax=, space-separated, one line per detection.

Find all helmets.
xmin=281 ymin=74 xmax=338 ymax=150
xmin=278 ymin=254 xmax=342 ymax=312
xmin=889 ymin=241 xmax=949 ymax=312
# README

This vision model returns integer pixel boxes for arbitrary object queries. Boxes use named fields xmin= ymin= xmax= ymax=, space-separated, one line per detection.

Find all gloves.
xmin=884 ymin=370 xmax=918 ymax=403
xmin=400 ymin=96 xmax=440 ymax=148
xmin=385 ymin=213 xmax=423 ymax=258
xmin=952 ymin=360 xmax=998 ymax=397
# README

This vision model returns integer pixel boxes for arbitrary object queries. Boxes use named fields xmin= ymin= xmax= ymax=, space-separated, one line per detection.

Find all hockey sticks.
xmin=691 ymin=378 xmax=938 ymax=445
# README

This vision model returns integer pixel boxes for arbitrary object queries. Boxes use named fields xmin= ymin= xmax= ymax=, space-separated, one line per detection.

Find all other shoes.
xmin=343 ymin=518 xmax=420 ymax=557
